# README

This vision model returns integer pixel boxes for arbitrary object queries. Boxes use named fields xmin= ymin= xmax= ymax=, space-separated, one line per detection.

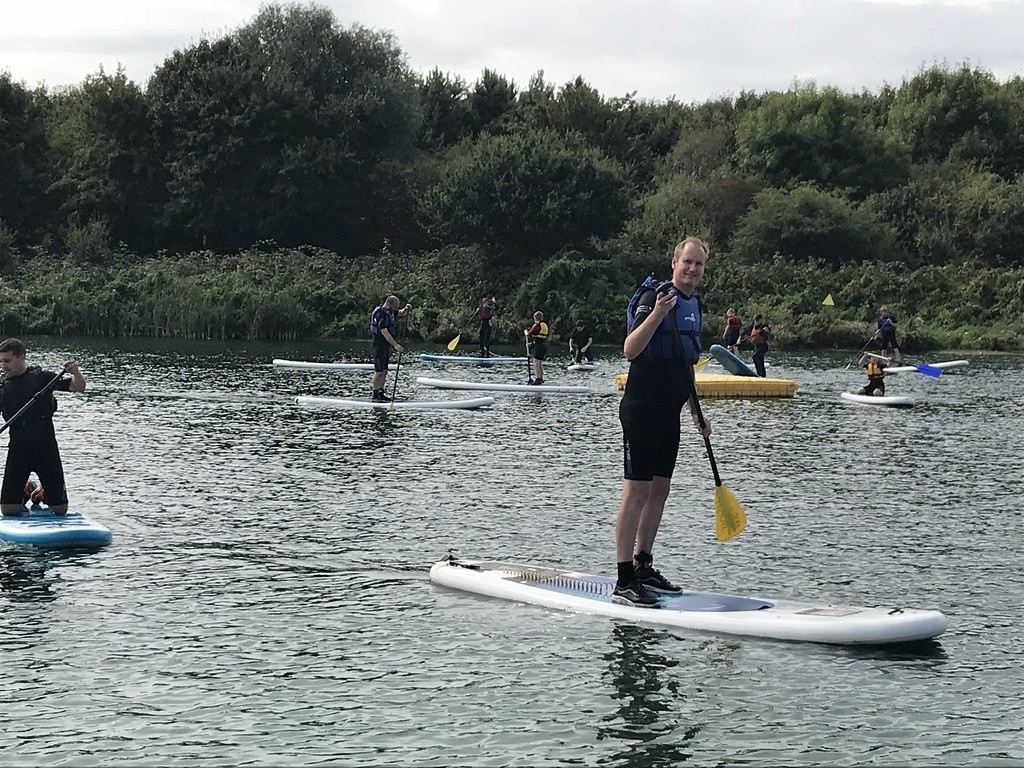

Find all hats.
xmin=753 ymin=313 xmax=762 ymax=320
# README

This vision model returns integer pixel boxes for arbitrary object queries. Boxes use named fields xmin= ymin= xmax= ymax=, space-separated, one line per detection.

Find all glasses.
xmin=576 ymin=325 xmax=583 ymax=327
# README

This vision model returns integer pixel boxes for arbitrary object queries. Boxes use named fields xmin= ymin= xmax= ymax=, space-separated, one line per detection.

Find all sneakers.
xmin=637 ymin=570 xmax=683 ymax=594
xmin=612 ymin=579 xmax=662 ymax=609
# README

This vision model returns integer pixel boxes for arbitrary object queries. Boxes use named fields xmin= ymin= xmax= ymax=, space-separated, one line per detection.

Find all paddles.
xmin=867 ymin=352 xmax=944 ymax=377
xmin=0 ymin=364 xmax=68 ymax=436
xmin=657 ymin=280 xmax=746 ymax=542
xmin=391 ymin=306 xmax=410 ymax=410
xmin=845 ymin=316 xmax=890 ymax=371
xmin=525 ymin=335 xmax=534 ymax=384
xmin=447 ymin=294 xmax=490 ymax=351
xmin=694 ymin=331 xmax=762 ymax=372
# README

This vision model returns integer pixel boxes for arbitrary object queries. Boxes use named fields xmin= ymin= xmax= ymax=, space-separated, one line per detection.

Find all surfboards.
xmin=0 ymin=501 xmax=114 ymax=550
xmin=272 ymin=358 xmax=405 ymax=372
xmin=415 ymin=376 xmax=590 ymax=393
xmin=567 ymin=360 xmax=602 ymax=370
xmin=883 ymin=359 xmax=969 ymax=373
xmin=709 ymin=344 xmax=761 ymax=377
xmin=293 ymin=396 xmax=495 ymax=410
xmin=419 ymin=353 xmax=529 ymax=362
xmin=425 ymin=555 xmax=946 ymax=647
xmin=840 ymin=391 xmax=917 ymax=406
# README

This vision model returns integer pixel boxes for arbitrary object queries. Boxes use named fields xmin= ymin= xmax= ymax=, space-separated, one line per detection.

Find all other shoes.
xmin=896 ymin=363 xmax=901 ymax=367
xmin=31 ymin=486 xmax=44 ymax=505
xmin=372 ymin=392 xmax=393 ymax=402
xmin=25 ymin=480 xmax=37 ymax=493
xmin=478 ymin=354 xmax=485 ymax=357
xmin=483 ymin=354 xmax=491 ymax=358
xmin=532 ymin=377 xmax=544 ymax=385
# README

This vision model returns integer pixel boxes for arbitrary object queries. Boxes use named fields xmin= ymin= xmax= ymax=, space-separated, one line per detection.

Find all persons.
xmin=875 ymin=306 xmax=901 ymax=367
xmin=480 ymin=297 xmax=496 ymax=358
xmin=524 ymin=311 xmax=549 ymax=385
xmin=569 ymin=320 xmax=593 ymax=364
xmin=857 ymin=350 xmax=892 ymax=396
xmin=751 ymin=313 xmax=770 ymax=378
xmin=611 ymin=238 xmax=711 ymax=607
xmin=0 ymin=339 xmax=86 ymax=518
xmin=370 ymin=295 xmax=412 ymax=400
xmin=723 ymin=308 xmax=743 ymax=358
